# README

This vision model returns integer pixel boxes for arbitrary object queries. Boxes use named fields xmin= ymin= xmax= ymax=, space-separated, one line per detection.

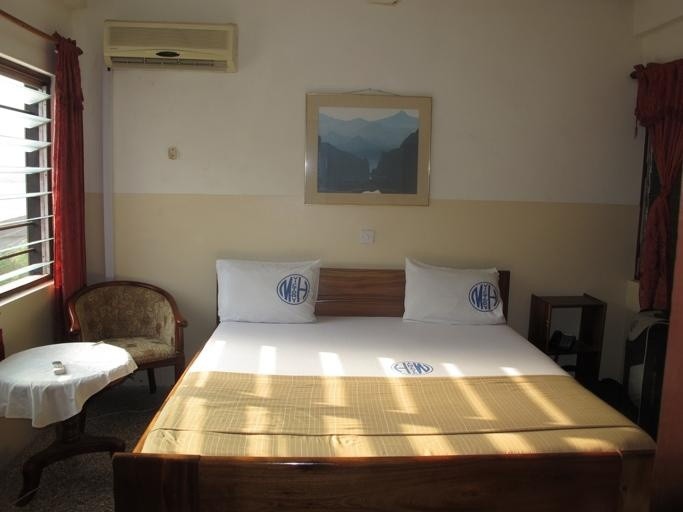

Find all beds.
xmin=111 ymin=268 xmax=658 ymax=512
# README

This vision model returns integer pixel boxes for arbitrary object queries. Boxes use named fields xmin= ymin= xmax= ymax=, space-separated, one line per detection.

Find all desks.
xmin=0 ymin=340 xmax=132 ymax=510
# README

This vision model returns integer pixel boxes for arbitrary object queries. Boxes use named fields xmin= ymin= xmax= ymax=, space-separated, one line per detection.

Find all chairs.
xmin=65 ymin=280 xmax=188 ymax=393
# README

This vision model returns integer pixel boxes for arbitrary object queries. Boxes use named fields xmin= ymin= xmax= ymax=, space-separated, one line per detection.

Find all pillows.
xmin=401 ymin=256 xmax=506 ymax=326
xmin=215 ymin=257 xmax=321 ymax=326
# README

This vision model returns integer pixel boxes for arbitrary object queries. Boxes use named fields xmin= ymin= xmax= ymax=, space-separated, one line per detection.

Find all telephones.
xmin=548 ymin=330 xmax=576 ymax=353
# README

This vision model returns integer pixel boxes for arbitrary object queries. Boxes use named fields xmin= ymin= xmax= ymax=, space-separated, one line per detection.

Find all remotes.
xmin=50 ymin=360 xmax=65 ymax=375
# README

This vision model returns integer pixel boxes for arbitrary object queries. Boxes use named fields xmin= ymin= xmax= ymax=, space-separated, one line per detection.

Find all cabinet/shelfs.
xmin=527 ymin=295 xmax=608 ymax=379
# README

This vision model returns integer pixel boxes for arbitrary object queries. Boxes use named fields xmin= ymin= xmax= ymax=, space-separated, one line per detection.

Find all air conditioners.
xmin=102 ymin=19 xmax=239 ymax=73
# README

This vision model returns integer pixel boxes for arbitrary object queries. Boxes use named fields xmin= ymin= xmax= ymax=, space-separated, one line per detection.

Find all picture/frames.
xmin=303 ymin=91 xmax=434 ymax=207
